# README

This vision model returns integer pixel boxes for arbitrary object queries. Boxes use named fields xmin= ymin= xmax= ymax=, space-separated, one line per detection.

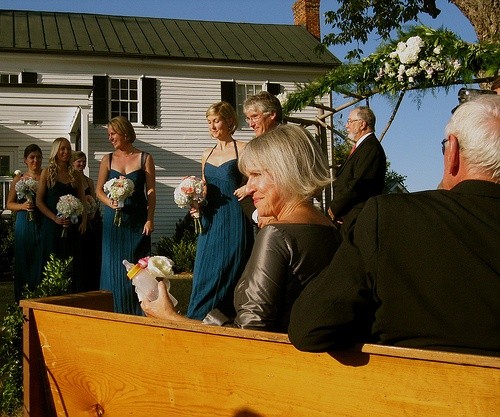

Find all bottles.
xmin=122 ymin=258 xmax=178 ymax=306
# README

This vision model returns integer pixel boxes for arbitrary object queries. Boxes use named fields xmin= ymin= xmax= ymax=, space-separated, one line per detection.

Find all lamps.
xmin=265 ymin=82 xmax=281 ymax=95
xmin=17 ymin=72 xmax=37 ymax=83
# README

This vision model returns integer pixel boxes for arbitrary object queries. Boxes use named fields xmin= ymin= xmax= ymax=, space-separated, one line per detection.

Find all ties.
xmin=347 ymin=144 xmax=357 ymax=160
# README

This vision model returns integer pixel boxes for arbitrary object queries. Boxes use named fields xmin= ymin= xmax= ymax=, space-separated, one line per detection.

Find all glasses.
xmin=346 ymin=118 xmax=362 ymax=125
xmin=244 ymin=113 xmax=264 ymax=122
xmin=441 ymin=138 xmax=449 ymax=155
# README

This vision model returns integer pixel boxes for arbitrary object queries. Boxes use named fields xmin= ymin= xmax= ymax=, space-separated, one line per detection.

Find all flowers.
xmin=174 ymin=174 xmax=207 ymax=234
xmin=15 ymin=169 xmax=40 ymax=222
xmin=148 ymin=255 xmax=175 ymax=279
xmin=104 ymin=176 xmax=135 ymax=228
xmin=376 ymin=32 xmax=465 ymax=90
xmin=87 ymin=195 xmax=98 ymax=219
xmin=56 ymin=195 xmax=83 ymax=238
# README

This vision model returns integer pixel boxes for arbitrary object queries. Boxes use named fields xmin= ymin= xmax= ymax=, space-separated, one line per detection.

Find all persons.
xmin=328 ymin=106 xmax=386 ymax=222
xmin=491 ymin=76 xmax=500 ymax=95
xmin=6 ymin=137 xmax=100 ymax=304
xmin=288 ymin=95 xmax=500 ymax=358
xmin=186 ymin=102 xmax=249 ymax=320
xmin=243 ymin=91 xmax=283 ymax=136
xmin=141 ymin=124 xmax=343 ymax=331
xmin=96 ymin=117 xmax=155 ymax=316
